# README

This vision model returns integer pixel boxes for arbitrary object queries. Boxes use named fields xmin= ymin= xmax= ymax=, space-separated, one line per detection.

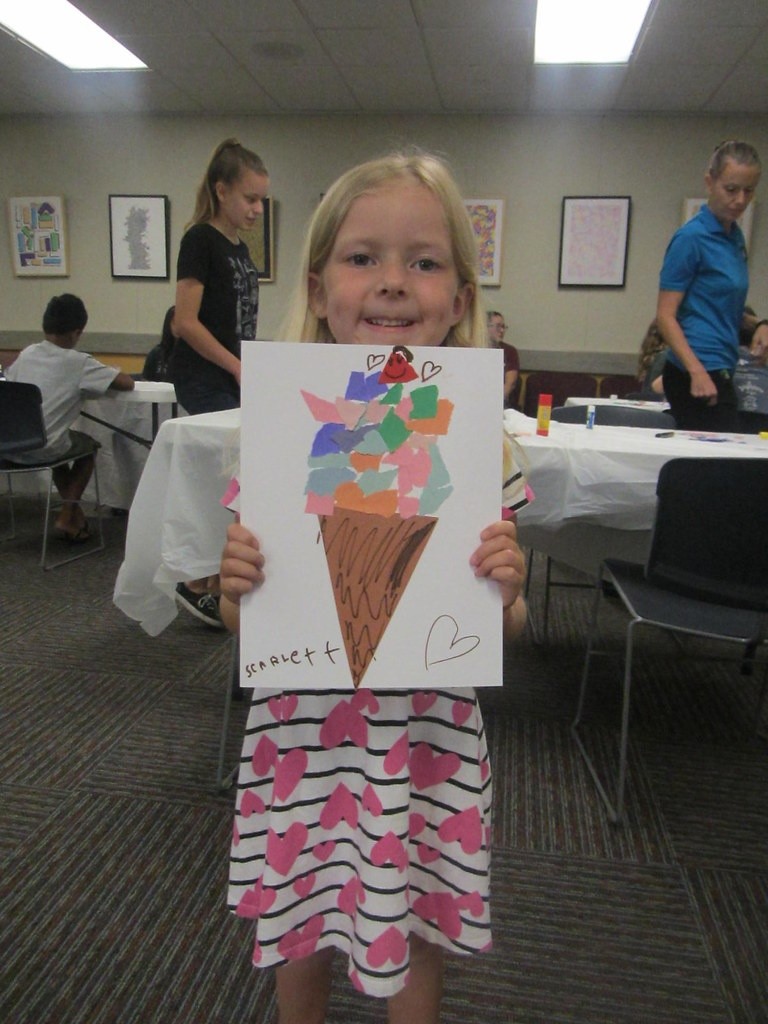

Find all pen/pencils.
xmin=655 ymin=432 xmax=675 ymax=438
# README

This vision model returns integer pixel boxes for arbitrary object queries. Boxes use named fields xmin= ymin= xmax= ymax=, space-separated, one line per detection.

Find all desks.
xmin=0 ymin=382 xmax=179 ymax=515
xmin=114 ymin=408 xmax=768 ymax=645
xmin=560 ymin=396 xmax=677 ymax=428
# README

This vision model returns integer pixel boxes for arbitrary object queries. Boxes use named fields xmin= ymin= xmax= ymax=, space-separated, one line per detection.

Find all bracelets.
xmin=754 ymin=318 xmax=768 ymax=331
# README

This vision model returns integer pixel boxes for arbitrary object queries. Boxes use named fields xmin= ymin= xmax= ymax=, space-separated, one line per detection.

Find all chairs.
xmin=0 ymin=379 xmax=105 ymax=571
xmin=571 ymin=455 xmax=768 ymax=826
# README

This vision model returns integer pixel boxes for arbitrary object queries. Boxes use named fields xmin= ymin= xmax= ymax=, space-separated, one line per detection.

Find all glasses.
xmin=490 ymin=323 xmax=508 ymax=329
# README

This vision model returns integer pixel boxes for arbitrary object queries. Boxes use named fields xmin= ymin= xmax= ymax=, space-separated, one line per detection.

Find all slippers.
xmin=52 ymin=520 xmax=92 ymax=545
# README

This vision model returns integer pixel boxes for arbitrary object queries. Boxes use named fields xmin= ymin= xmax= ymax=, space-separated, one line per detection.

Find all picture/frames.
xmin=556 ymin=195 xmax=633 ymax=288
xmin=108 ymin=194 xmax=171 ymax=279
xmin=235 ymin=194 xmax=275 ymax=284
xmin=680 ymin=192 xmax=754 ymax=259
xmin=459 ymin=192 xmax=507 ymax=290
xmin=8 ymin=191 xmax=71 ymax=278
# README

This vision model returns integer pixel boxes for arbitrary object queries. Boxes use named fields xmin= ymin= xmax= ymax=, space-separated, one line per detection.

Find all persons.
xmin=655 ymin=140 xmax=768 ymax=434
xmin=171 ymin=138 xmax=267 ymax=627
xmin=487 ymin=311 xmax=519 ymax=410
xmin=143 ymin=305 xmax=181 ymax=382
xmin=4 ymin=294 xmax=134 ymax=541
xmin=635 ymin=306 xmax=757 ymax=405
xmin=218 ymin=153 xmax=529 ymax=1024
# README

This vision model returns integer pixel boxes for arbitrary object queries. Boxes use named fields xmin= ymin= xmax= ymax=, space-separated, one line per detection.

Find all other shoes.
xmin=112 ymin=508 xmax=126 ymax=515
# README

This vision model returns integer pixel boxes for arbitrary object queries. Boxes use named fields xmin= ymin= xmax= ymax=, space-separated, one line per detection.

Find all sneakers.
xmin=176 ymin=583 xmax=224 ymax=628
